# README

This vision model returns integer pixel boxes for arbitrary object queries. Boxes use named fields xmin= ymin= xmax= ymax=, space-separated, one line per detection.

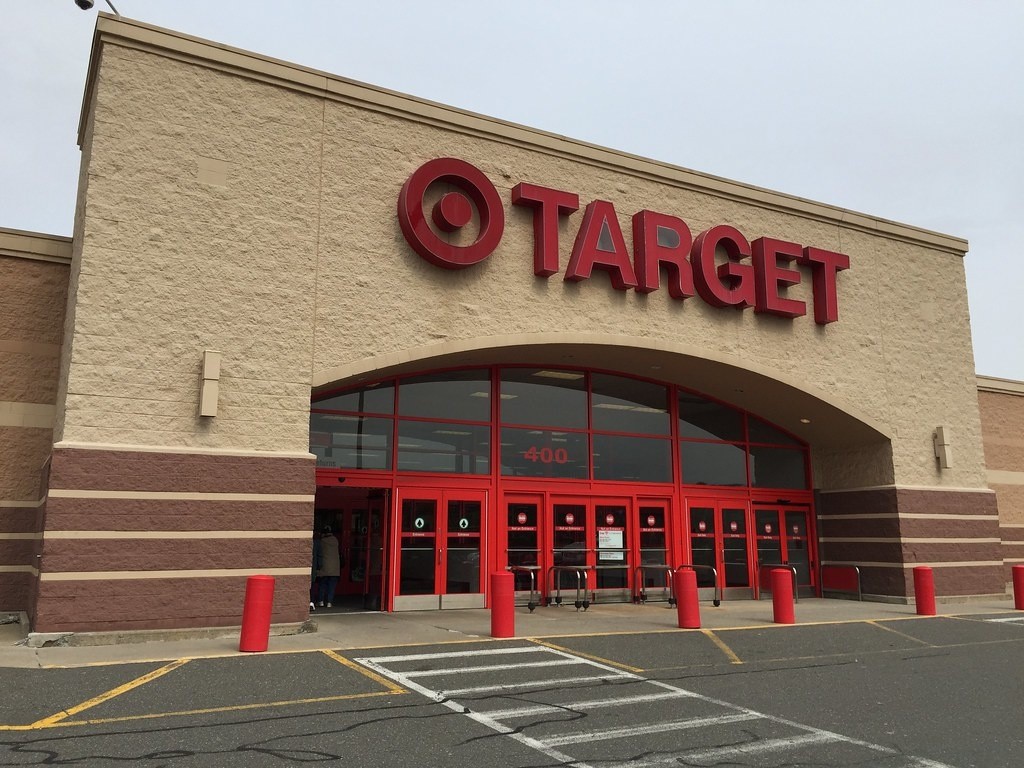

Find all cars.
xmin=554 ymin=541 xmax=586 ymax=564
